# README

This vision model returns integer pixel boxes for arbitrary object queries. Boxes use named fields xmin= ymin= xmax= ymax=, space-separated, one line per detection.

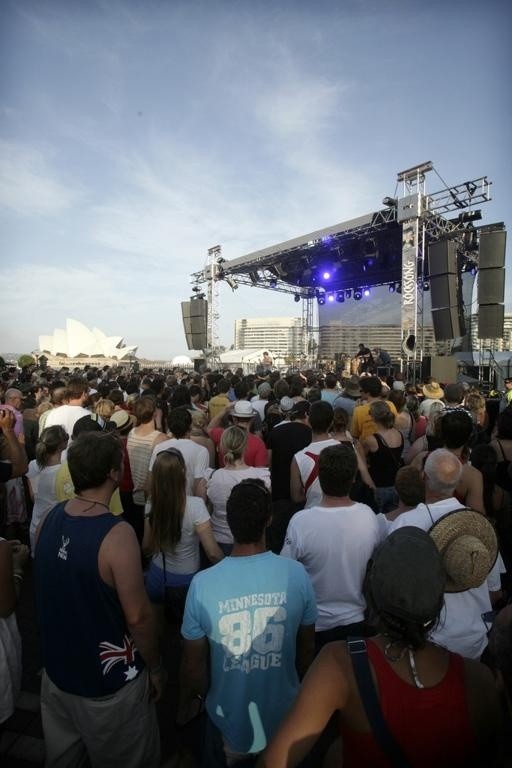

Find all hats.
xmin=228 ymin=400 xmax=258 ymax=418
xmin=427 ymin=506 xmax=501 ymax=594
xmin=345 ymin=374 xmax=365 ymax=397
xmin=366 ymin=523 xmax=446 ymax=621
xmin=280 ymin=395 xmax=296 ymax=414
xmin=5 ymin=388 xmax=28 ymax=400
xmin=422 ymin=381 xmax=445 ymax=399
xmin=108 ymin=409 xmax=138 ymax=433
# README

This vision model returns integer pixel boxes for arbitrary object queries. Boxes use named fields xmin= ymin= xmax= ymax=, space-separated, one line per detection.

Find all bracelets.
xmin=192 ymin=692 xmax=207 ymax=702
xmin=146 ymin=662 xmax=168 ymax=675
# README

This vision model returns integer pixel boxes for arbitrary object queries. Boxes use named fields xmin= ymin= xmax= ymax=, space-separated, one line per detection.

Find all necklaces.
xmin=72 ymin=496 xmax=112 ymax=514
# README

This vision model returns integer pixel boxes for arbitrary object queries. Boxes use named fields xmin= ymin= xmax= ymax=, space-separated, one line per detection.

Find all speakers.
xmin=478 ymin=304 xmax=504 ymax=339
xmin=428 ymin=240 xmax=459 ymax=275
xmin=430 ymin=308 xmax=461 ymax=342
xmin=477 ymin=268 xmax=506 ymax=304
xmin=180 ymin=301 xmax=208 ymax=350
xmin=429 ymin=274 xmax=458 ymax=308
xmin=422 ymin=355 xmax=458 ymax=384
xmin=194 ymin=359 xmax=204 ymax=371
xmin=477 ymin=231 xmax=507 ymax=269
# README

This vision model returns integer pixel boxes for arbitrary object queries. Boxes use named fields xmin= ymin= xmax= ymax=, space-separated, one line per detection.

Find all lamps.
xmin=382 ymin=195 xmax=397 ymax=207
xmin=191 ymin=285 xmax=202 ymax=292
xmin=222 ymin=233 xmax=429 ymax=305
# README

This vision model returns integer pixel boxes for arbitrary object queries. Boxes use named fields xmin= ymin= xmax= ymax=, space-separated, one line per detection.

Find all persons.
xmin=255 ymin=525 xmax=499 ymax=768
xmin=179 ymin=476 xmax=325 ymax=767
xmin=0 ymin=537 xmax=28 ymax=723
xmin=31 ymin=430 xmax=170 ymax=768
